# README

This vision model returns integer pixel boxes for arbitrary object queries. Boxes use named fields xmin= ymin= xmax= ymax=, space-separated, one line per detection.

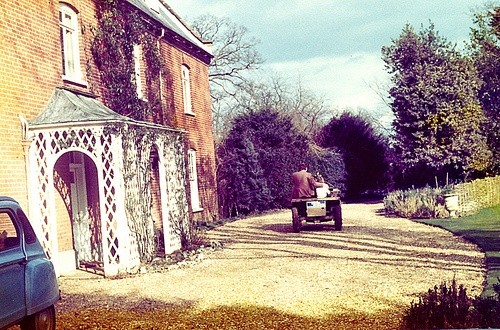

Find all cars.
xmin=0 ymin=197 xmax=60 ymax=330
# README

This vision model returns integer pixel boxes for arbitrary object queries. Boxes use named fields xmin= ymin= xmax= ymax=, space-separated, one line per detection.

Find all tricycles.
xmin=292 ymin=181 xmax=343 ymax=230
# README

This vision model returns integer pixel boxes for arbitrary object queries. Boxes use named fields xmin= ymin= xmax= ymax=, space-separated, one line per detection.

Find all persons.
xmin=315 ymin=172 xmax=330 ymax=198
xmin=290 ymin=164 xmax=322 ymax=199
